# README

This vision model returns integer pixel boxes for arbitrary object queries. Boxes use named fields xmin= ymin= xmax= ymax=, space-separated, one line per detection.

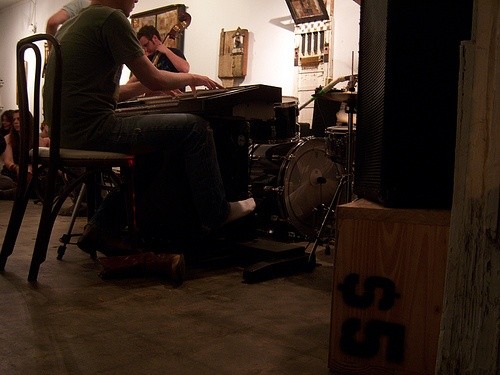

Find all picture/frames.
xmin=285 ymin=0 xmax=330 ymax=25
xmin=130 ymin=4 xmax=186 ymax=54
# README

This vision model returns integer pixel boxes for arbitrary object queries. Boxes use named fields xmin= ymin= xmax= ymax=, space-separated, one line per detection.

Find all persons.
xmin=44 ymin=0 xmax=257 ymax=258
xmin=0 ymin=0 xmax=190 ymax=201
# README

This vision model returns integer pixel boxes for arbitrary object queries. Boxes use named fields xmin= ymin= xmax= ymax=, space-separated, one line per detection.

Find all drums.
xmin=240 ymin=134 xmax=355 ymax=244
xmin=252 ymin=95 xmax=300 ymax=144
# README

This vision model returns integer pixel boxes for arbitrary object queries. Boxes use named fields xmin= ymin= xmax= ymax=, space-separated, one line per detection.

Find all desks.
xmin=329 ymin=198 xmax=450 ymax=375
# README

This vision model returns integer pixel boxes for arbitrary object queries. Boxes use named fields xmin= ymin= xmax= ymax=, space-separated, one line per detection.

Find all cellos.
xmin=131 ymin=12 xmax=192 ymax=102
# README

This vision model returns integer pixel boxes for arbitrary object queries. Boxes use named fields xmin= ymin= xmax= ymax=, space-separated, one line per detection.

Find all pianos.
xmin=86 ymin=83 xmax=318 ymax=283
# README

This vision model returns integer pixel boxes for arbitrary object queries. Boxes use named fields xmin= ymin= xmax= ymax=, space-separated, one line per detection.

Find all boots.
xmin=77 ymin=219 xmax=137 ymax=256
xmin=94 ymin=250 xmax=185 ymax=283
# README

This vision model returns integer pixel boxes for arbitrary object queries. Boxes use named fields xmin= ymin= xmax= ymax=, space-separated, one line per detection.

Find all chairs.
xmin=0 ymin=33 xmax=139 ymax=281
